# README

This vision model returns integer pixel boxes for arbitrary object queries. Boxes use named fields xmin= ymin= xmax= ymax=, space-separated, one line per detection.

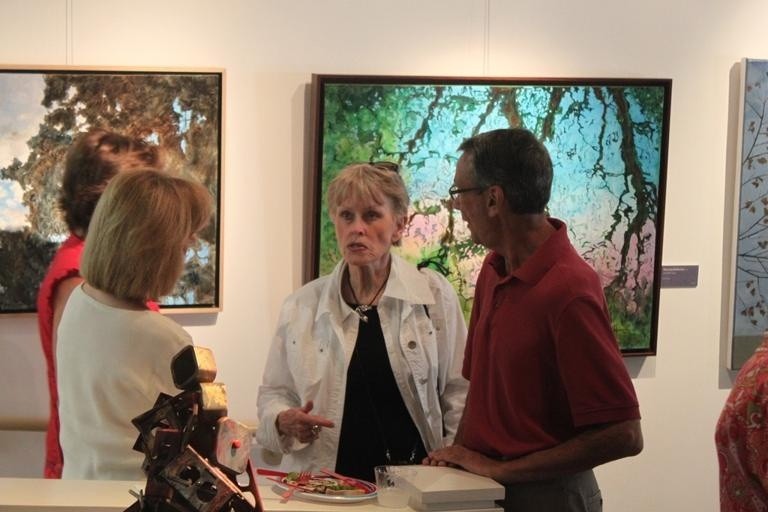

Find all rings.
xmin=312 ymin=425 xmax=319 ymax=435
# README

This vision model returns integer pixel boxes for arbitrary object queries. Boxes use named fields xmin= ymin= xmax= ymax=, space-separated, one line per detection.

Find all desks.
xmin=1 ymin=476 xmax=505 ymax=512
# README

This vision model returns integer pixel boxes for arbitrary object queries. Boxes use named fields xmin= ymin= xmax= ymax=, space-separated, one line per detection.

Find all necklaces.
xmin=347 ymin=269 xmax=390 ymax=324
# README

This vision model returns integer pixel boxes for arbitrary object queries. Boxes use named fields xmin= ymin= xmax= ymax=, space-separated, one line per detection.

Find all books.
xmin=387 ymin=465 xmax=505 ymax=512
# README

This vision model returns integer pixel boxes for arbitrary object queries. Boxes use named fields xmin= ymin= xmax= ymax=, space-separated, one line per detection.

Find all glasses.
xmin=449 ymin=184 xmax=479 ymax=200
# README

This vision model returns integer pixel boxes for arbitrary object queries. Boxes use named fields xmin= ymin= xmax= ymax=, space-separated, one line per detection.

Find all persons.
xmin=38 ymin=131 xmax=165 ymax=479
xmin=255 ymin=160 xmax=470 ymax=486
xmin=55 ymin=167 xmax=215 ymax=479
xmin=714 ymin=328 xmax=768 ymax=512
xmin=423 ymin=128 xmax=643 ymax=512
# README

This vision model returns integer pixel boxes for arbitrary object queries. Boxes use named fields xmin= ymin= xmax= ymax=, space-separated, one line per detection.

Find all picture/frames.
xmin=0 ymin=64 xmax=227 ymax=320
xmin=312 ymin=72 xmax=672 ymax=357
xmin=727 ymin=59 xmax=768 ymax=372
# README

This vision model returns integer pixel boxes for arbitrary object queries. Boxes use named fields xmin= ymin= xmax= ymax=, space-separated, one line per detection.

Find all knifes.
xmin=320 ymin=466 xmax=370 ymax=492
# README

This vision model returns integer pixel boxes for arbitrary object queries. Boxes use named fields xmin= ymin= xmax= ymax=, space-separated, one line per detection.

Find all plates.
xmin=277 ymin=473 xmax=380 ymax=502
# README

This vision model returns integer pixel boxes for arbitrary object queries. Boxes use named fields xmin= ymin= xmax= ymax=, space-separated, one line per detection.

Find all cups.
xmin=374 ymin=465 xmax=414 ymax=507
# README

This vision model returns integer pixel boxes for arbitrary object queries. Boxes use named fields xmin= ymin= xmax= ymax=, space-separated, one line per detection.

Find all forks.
xmin=280 ymin=468 xmax=312 ymax=504
